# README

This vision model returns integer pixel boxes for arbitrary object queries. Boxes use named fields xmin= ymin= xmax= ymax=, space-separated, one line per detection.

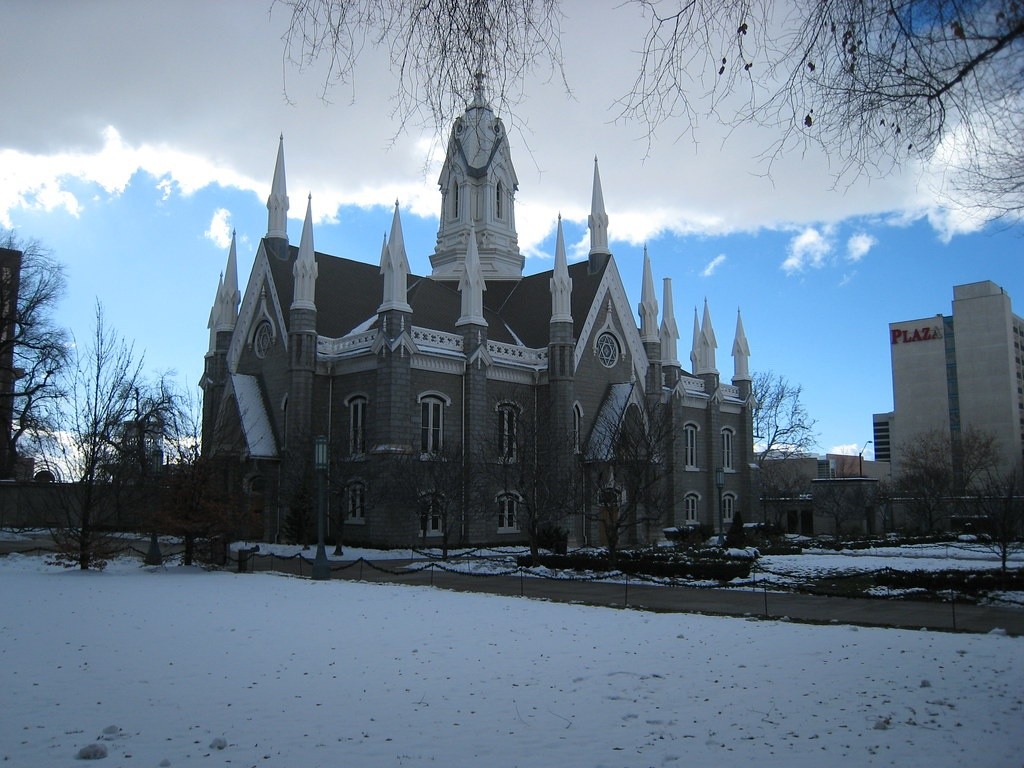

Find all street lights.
xmin=310 ymin=434 xmax=331 ymax=581
xmin=146 ymin=448 xmax=166 ymax=563
xmin=858 ymin=440 xmax=873 ymax=477
xmin=715 ymin=467 xmax=727 ymax=545
